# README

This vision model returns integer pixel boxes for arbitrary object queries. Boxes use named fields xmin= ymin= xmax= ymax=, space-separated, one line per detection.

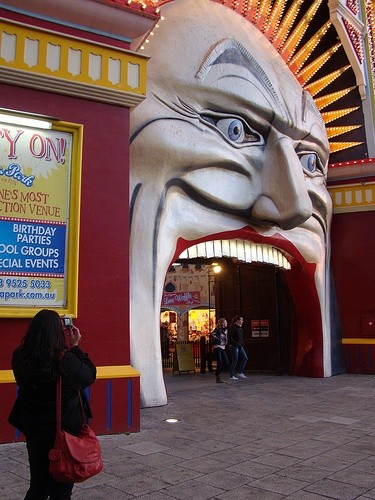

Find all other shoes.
xmin=238 ymin=373 xmax=247 ymax=379
xmin=229 ymin=376 xmax=238 ymax=380
xmin=216 ymin=377 xmax=224 ymax=383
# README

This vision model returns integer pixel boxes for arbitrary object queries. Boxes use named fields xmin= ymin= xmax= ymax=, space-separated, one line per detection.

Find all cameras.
xmin=61 ymin=316 xmax=73 ymax=328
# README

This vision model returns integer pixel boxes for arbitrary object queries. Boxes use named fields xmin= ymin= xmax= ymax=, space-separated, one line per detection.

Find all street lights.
xmin=207 ymin=262 xmax=222 ymax=373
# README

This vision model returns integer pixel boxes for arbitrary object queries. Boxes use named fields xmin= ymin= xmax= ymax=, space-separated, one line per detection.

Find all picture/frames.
xmin=0 ymin=110 xmax=85 ymax=319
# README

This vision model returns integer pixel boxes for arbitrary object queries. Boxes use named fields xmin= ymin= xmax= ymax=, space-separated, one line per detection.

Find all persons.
xmin=210 ymin=318 xmax=229 ymax=383
xmin=7 ymin=309 xmax=97 ymax=500
xmin=228 ymin=315 xmax=248 ymax=380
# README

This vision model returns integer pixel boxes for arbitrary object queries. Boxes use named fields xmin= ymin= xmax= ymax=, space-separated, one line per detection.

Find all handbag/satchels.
xmin=49 ymin=426 xmax=104 ymax=484
